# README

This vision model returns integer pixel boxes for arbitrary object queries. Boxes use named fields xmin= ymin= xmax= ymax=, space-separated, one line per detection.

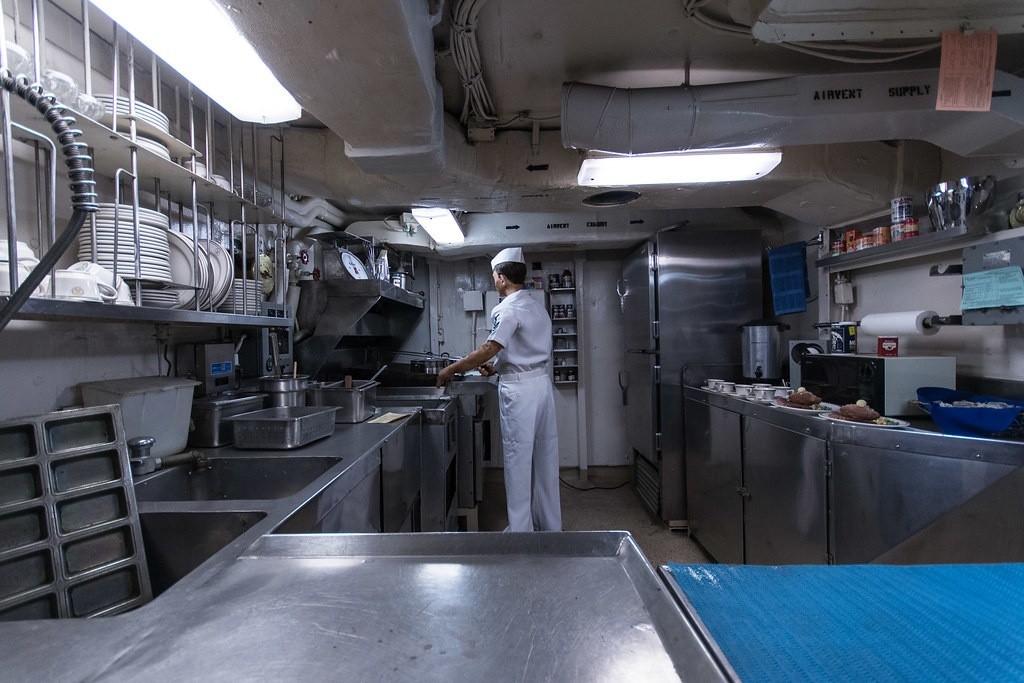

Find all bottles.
xmin=552 ymin=304 xmax=573 ymax=318
xmin=558 ymin=339 xmax=570 ymax=349
xmin=558 ymin=328 xmax=564 ymax=334
xmin=392 ymin=274 xmax=405 ymax=289
xmin=555 ymin=370 xmax=574 ymax=381
xmin=549 ymin=266 xmax=572 ymax=288
xmin=554 ymin=356 xmax=566 ymax=366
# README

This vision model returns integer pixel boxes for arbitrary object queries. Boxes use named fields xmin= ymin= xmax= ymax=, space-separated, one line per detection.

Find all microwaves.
xmin=800 ymin=352 xmax=956 ymax=416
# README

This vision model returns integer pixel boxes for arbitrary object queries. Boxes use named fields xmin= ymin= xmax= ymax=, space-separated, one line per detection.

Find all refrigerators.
xmin=617 ymin=219 xmax=767 ymax=525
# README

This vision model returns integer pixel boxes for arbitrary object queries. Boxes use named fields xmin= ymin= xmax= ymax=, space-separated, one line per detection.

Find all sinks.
xmin=373 ymin=396 xmax=446 ymax=411
xmin=382 ymin=384 xmax=446 ymax=397
xmin=132 ymin=454 xmax=345 ymax=500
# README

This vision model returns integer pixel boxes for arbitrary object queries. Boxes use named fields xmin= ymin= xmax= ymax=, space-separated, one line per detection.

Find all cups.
xmin=706 ymin=379 xmax=795 ymax=399
xmin=184 ymin=161 xmax=217 ymax=185
xmin=212 ymin=174 xmax=238 ymax=197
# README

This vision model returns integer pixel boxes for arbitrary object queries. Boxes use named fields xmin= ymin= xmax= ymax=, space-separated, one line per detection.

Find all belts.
xmin=498 ymin=368 xmax=548 ymax=381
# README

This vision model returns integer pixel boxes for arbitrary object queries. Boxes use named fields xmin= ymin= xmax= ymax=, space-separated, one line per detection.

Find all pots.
xmin=738 ymin=323 xmax=791 ymax=381
xmin=410 ymin=351 xmax=463 ymax=377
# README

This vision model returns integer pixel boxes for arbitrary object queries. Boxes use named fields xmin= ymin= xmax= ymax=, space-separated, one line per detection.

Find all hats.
xmin=490 ymin=246 xmax=527 ymax=271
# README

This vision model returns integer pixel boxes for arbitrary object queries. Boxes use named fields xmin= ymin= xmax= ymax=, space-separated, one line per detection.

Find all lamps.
xmin=575 ymin=147 xmax=784 ymax=189
xmin=409 ymin=206 xmax=466 ymax=246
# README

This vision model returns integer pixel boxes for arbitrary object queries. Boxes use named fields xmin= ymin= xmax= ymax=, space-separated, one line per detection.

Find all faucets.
xmin=754 ymin=366 xmax=763 ymax=380
xmin=127 ymin=436 xmax=210 ymax=477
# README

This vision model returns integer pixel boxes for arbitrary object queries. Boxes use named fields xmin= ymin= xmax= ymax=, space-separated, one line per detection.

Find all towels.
xmin=767 ymin=239 xmax=813 ymax=318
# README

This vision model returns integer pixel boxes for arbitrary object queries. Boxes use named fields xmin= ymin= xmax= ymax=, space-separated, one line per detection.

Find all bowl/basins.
xmin=925 ymin=174 xmax=996 ymax=230
xmin=523 ymin=282 xmax=534 ymax=289
xmin=0 ymin=260 xmax=103 ymax=302
xmin=0 ymin=39 xmax=106 ymax=122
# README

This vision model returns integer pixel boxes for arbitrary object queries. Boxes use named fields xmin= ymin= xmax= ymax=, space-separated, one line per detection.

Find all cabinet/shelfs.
xmin=1 ymin=0 xmax=293 ymax=328
xmin=547 ymin=287 xmax=577 ymax=385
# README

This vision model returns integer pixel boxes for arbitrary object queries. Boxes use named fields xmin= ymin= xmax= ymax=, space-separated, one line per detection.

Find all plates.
xmin=94 ymin=94 xmax=169 ymax=134
xmin=218 ymin=279 xmax=265 ymax=315
xmin=770 ymin=400 xmax=835 ymax=414
xmin=818 ymin=412 xmax=910 ymax=427
xmin=701 ymin=386 xmax=775 ymax=404
xmin=77 ymin=203 xmax=235 ymax=308
xmin=116 ymin=131 xmax=170 ymax=162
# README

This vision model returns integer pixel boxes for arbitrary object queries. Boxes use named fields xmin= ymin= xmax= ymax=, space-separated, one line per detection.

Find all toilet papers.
xmin=860 ymin=309 xmax=941 ymax=337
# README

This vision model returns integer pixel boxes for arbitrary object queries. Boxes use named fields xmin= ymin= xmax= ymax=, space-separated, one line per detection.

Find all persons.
xmin=437 ymin=247 xmax=561 ymax=532
